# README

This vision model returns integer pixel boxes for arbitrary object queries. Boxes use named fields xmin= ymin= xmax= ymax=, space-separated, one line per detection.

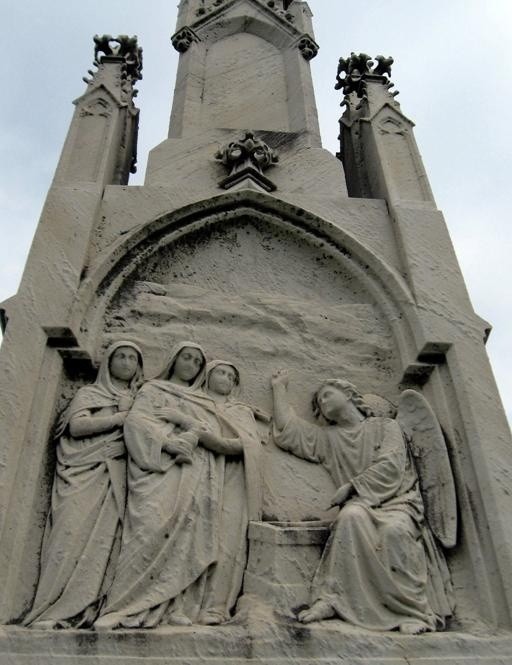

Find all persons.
xmin=192 ymin=360 xmax=260 ymax=625
xmin=90 ymin=341 xmax=225 ymax=631
xmin=18 ymin=341 xmax=144 ymax=631
xmin=270 ymin=368 xmax=456 ymax=635
